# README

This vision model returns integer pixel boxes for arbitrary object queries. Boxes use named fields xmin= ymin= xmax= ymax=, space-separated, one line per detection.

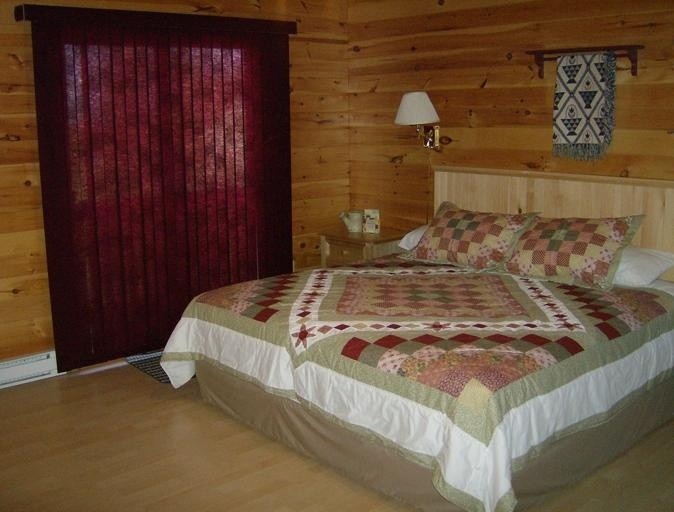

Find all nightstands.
xmin=319 ymin=224 xmax=405 ymax=269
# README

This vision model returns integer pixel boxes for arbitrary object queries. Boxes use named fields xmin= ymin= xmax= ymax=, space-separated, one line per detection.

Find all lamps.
xmin=395 ymin=89 xmax=441 ymax=151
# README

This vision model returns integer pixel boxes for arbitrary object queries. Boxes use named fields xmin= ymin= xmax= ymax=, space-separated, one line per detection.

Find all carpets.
xmin=126 ymin=347 xmax=196 ymax=386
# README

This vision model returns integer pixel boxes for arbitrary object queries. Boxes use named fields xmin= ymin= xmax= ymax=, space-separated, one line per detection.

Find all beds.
xmin=192 ymin=163 xmax=674 ymax=509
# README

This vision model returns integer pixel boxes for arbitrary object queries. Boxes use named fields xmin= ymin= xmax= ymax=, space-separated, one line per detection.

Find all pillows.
xmin=400 ymin=201 xmax=674 ymax=293
